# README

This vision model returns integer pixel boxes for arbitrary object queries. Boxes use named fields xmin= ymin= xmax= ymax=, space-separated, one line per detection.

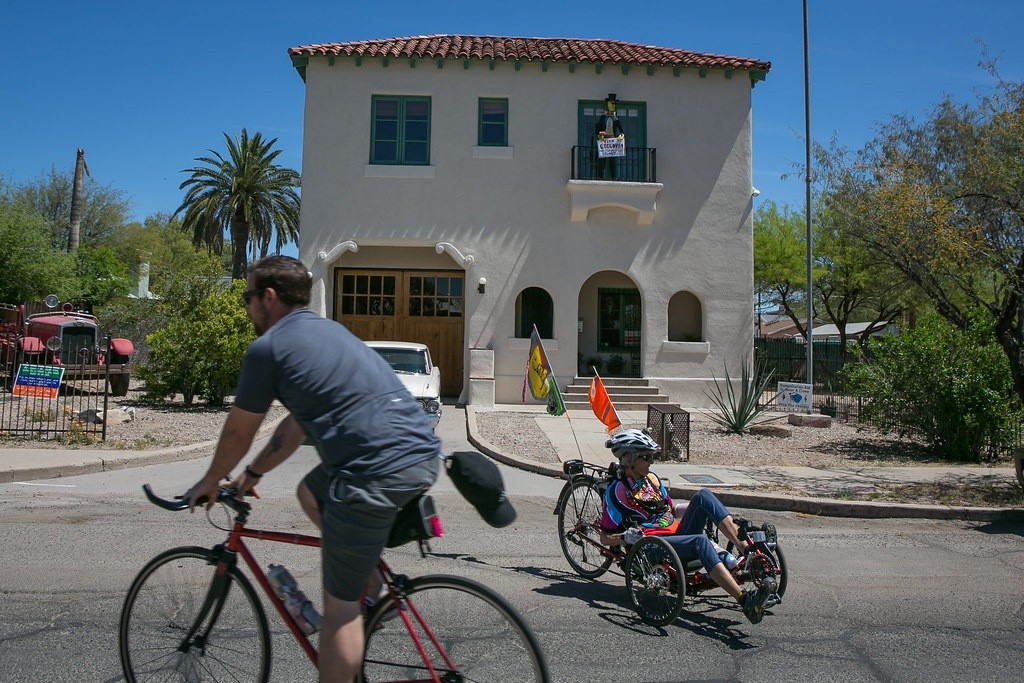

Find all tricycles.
xmin=553 ymin=460 xmax=788 ymax=627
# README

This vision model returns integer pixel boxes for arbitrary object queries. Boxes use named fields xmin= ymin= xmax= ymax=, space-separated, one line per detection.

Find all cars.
xmin=363 ymin=341 xmax=443 ymax=434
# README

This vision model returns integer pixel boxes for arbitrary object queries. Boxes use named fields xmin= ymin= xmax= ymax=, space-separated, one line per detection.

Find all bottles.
xmin=359 ymin=565 xmax=382 ymax=625
xmin=266 ymin=563 xmax=322 ymax=635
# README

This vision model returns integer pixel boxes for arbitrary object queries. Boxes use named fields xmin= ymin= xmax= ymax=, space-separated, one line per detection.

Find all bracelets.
xmin=245 ymin=464 xmax=263 ymax=479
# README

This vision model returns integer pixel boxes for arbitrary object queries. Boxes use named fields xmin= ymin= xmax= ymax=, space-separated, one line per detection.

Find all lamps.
xmin=478 ymin=278 xmax=486 ymax=294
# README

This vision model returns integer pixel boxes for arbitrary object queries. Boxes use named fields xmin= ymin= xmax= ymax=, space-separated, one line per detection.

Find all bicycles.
xmin=119 ymin=484 xmax=549 ymax=683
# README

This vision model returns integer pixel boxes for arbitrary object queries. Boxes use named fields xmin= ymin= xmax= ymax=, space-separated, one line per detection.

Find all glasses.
xmin=243 ymin=288 xmax=265 ymax=304
xmin=637 ymin=453 xmax=653 ymax=461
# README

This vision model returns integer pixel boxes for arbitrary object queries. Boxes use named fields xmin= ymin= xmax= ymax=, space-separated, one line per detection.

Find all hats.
xmin=444 ymin=451 xmax=517 ymax=528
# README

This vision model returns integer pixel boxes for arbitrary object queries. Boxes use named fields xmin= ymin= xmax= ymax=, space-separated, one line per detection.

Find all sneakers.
xmin=761 ymin=522 xmax=777 ymax=553
xmin=741 ymin=577 xmax=778 ymax=624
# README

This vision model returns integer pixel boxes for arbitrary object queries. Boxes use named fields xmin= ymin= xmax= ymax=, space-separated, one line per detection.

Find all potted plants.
xmin=586 ymin=352 xmax=625 ymax=375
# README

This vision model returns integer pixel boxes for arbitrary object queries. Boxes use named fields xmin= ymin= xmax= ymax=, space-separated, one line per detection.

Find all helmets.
xmin=611 ymin=429 xmax=662 ymax=459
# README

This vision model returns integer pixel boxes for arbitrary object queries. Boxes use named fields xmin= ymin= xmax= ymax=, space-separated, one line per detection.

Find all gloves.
xmin=617 ymin=527 xmax=647 ymax=545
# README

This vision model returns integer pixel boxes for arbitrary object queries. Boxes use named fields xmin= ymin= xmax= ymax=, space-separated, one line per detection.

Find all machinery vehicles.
xmin=0 ymin=295 xmax=134 ymax=396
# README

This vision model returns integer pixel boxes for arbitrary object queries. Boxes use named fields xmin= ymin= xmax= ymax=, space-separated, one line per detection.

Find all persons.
xmin=599 ymin=428 xmax=777 ymax=624
xmin=183 ymin=253 xmax=440 ymax=683
xmin=595 ymin=94 xmax=624 ymax=181
